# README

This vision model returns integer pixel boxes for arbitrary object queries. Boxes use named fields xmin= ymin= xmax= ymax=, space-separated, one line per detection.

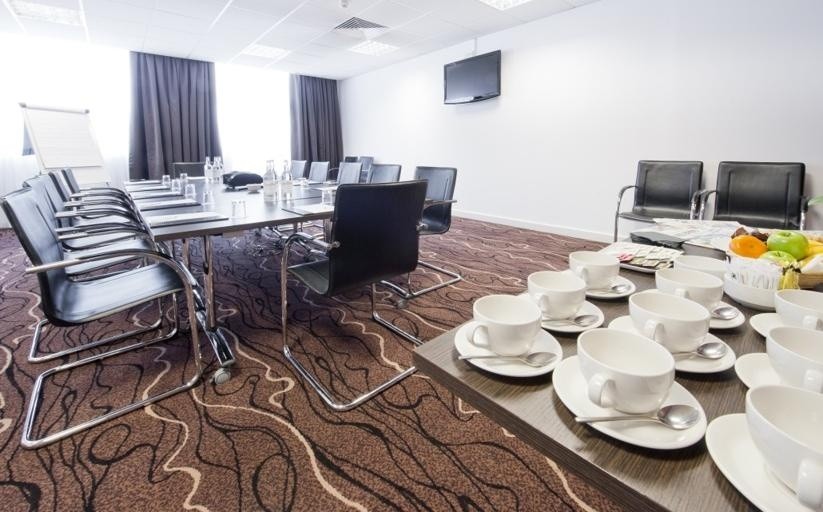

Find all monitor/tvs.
xmin=444 ymin=50 xmax=501 ymax=104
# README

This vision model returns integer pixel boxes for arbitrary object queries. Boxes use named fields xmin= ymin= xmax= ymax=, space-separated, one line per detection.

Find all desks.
xmin=123 ymin=166 xmax=339 ymax=368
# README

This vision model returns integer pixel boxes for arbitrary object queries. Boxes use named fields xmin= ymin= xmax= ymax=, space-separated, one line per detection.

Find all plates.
xmin=454 ymin=321 xmax=563 ymax=377
xmin=608 ymin=316 xmax=736 ymax=373
xmin=724 ymin=283 xmax=775 ymax=310
xmin=598 ymin=241 xmax=684 ymax=273
xmin=728 ymin=244 xmax=823 ymax=288
xmin=750 ymin=312 xmax=783 ymax=338
xmin=734 ymin=352 xmax=782 ymax=389
xmin=705 ymin=413 xmax=816 ymax=512
xmin=541 ymin=300 xmax=605 ymax=332
xmin=552 ymin=354 xmax=707 ymax=449
xmin=586 ymin=275 xmax=636 ymax=300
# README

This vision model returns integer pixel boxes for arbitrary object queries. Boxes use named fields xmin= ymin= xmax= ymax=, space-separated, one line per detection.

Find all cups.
xmin=301 ymin=180 xmax=309 ymax=189
xmin=322 ymin=189 xmax=332 ymax=207
xmin=528 ymin=271 xmax=587 ymax=320
xmin=656 ymin=269 xmax=725 ymax=314
xmin=766 ymin=326 xmax=823 ymax=393
xmin=172 ymin=179 xmax=181 ymax=191
xmin=628 ymin=292 xmax=711 ymax=354
xmin=467 ymin=293 xmax=542 ymax=357
xmin=745 ymin=384 xmax=823 ymax=511
xmin=247 ymin=184 xmax=260 ymax=192
xmin=673 ymin=255 xmax=728 ymax=283
xmin=569 ymin=251 xmax=620 ymax=289
xmin=162 ymin=174 xmax=171 ymax=187
xmin=184 ymin=185 xmax=195 ymax=195
xmin=231 ymin=201 xmax=246 ymax=219
xmin=577 ymin=327 xmax=676 ymax=414
xmin=179 ymin=173 xmax=188 ymax=183
xmin=202 ymin=191 xmax=213 ymax=205
xmin=775 ymin=289 xmax=823 ymax=330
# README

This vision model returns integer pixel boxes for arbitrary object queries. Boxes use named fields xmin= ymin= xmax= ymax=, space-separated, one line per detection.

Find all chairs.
xmin=22 ymin=176 xmax=179 ymax=364
xmin=366 ymin=162 xmax=402 ymax=184
xmin=61 ymin=168 xmax=137 ymax=220
xmin=698 ymin=160 xmax=808 ymax=232
xmin=359 ymin=156 xmax=373 ymax=182
xmin=344 ymin=156 xmax=358 ymax=162
xmin=337 ymin=162 xmax=363 ymax=184
xmin=613 ymin=160 xmax=703 ymax=243
xmin=309 ymin=161 xmax=330 ymax=182
xmin=47 ymin=169 xmax=138 ymax=226
xmin=290 ymin=160 xmax=309 ymax=179
xmin=279 ymin=180 xmax=429 ymax=412
xmin=380 ymin=166 xmax=460 ymax=299
xmin=0 ymin=187 xmax=205 ymax=450
xmin=35 ymin=173 xmax=156 ymax=280
xmin=173 ymin=163 xmax=215 ymax=180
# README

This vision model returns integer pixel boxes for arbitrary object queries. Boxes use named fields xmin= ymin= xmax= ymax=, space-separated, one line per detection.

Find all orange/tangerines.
xmin=729 ymin=235 xmax=768 ymax=259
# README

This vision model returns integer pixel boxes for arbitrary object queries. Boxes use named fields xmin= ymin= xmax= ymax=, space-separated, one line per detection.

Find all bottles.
xmin=263 ymin=160 xmax=275 ymax=199
xmin=271 ymin=160 xmax=278 ymax=195
xmin=281 ymin=160 xmax=293 ymax=196
xmin=204 ymin=157 xmax=212 ymax=184
xmin=212 ymin=157 xmax=220 ymax=184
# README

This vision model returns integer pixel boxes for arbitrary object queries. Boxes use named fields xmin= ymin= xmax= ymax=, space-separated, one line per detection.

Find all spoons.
xmin=542 ymin=314 xmax=599 ymax=327
xmin=458 ymin=352 xmax=558 ymax=367
xmin=587 ymin=284 xmax=631 ymax=294
xmin=711 ymin=306 xmax=739 ymax=320
xmin=672 ymin=342 xmax=728 ymax=359
xmin=575 ymin=404 xmax=701 ymax=430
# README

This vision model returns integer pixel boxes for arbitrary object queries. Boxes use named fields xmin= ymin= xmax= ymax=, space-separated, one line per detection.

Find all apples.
xmin=758 ymin=250 xmax=798 ymax=266
xmin=767 ymin=230 xmax=808 ymax=260
